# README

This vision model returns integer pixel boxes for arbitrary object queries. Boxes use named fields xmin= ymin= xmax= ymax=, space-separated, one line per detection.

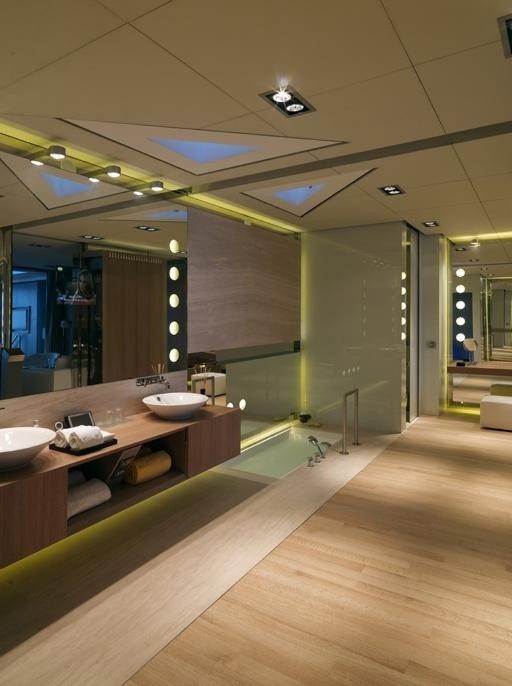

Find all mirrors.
xmin=187 ymin=340 xmax=301 ymax=441
xmin=0 ymin=149 xmax=188 ymax=402
xmin=446 ymin=237 xmax=512 ymax=408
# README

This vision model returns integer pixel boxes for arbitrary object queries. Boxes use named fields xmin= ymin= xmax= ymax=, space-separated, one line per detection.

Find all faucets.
xmin=0 ymin=405 xmax=6 ymax=413
xmin=159 ymin=379 xmax=172 ymax=392
xmin=308 ymin=435 xmax=324 ymax=461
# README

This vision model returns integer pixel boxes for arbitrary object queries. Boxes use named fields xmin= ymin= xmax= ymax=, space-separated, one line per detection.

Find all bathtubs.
xmin=225 ymin=419 xmax=345 ymax=485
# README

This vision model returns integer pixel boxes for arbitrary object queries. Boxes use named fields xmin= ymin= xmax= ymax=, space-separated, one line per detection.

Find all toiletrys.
xmin=34 ymin=418 xmax=40 ymax=427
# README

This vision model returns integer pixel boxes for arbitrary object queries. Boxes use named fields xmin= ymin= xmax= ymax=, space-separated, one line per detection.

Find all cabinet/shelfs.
xmin=0 ymin=404 xmax=242 ymax=571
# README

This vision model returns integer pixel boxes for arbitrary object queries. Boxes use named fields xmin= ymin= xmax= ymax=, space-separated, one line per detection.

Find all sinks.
xmin=141 ymin=392 xmax=209 ymax=420
xmin=0 ymin=426 xmax=57 ymax=466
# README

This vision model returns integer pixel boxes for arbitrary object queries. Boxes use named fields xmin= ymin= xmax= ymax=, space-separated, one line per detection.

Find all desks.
xmin=447 ymin=361 xmax=512 ymax=377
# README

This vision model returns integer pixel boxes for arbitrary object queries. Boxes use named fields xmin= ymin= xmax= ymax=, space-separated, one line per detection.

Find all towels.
xmin=53 ymin=424 xmax=174 ymax=524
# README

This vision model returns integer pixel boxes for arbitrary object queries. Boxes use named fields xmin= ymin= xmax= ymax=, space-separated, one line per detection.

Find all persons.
xmin=73 ymin=267 xmax=95 ymax=299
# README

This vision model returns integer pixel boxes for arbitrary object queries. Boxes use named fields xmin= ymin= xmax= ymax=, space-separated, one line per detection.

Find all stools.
xmin=190 ymin=372 xmax=227 ymax=397
xmin=479 ymin=395 xmax=512 ymax=431
xmin=489 ymin=383 xmax=512 ymax=396
